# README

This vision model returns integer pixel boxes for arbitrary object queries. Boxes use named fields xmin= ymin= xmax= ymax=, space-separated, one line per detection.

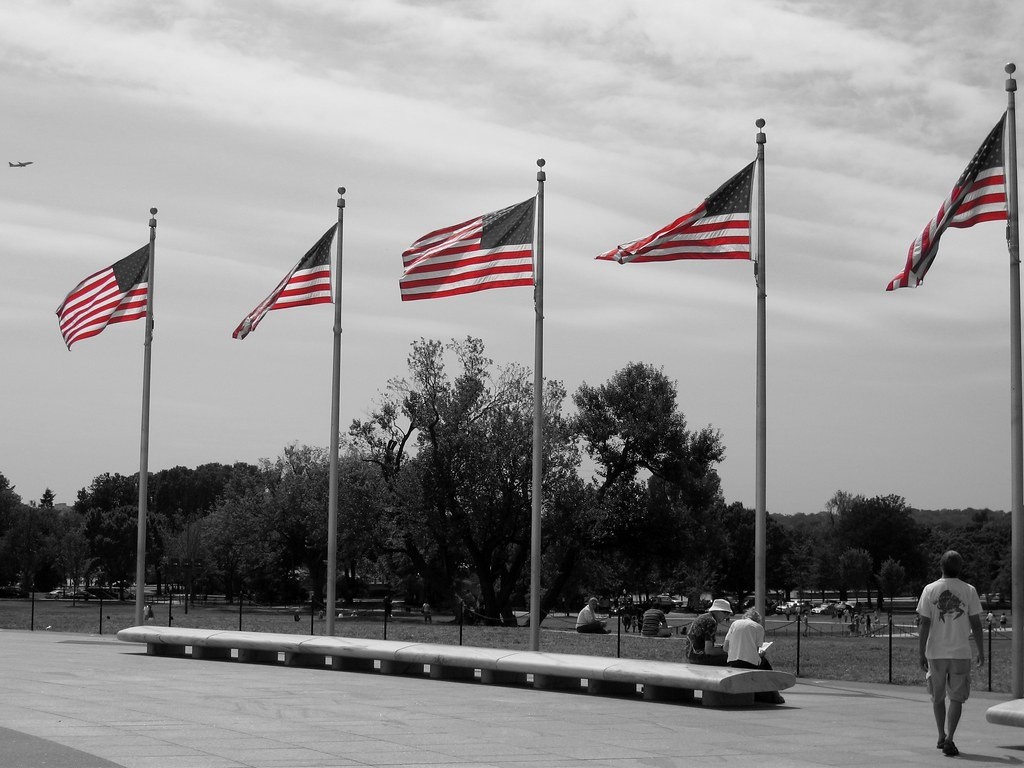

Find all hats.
xmin=709 ymin=599 xmax=732 ymax=612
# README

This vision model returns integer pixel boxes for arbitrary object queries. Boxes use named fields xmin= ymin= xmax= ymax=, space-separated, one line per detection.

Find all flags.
xmin=397 ymin=195 xmax=536 ymax=301
xmin=230 ymin=222 xmax=334 ymax=340
xmin=885 ymin=111 xmax=1009 ymax=292
xmin=593 ymin=160 xmax=756 ymax=266
xmin=52 ymin=241 xmax=150 ymax=352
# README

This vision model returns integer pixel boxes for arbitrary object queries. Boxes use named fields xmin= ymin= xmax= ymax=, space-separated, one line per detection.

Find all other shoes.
xmin=606 ymin=630 xmax=611 ymax=634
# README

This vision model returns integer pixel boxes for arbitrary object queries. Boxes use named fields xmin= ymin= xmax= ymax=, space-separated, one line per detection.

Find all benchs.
xmin=117 ymin=626 xmax=797 ymax=708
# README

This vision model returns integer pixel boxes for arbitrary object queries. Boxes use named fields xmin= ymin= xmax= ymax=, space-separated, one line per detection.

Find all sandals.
xmin=936 ymin=734 xmax=959 ymax=756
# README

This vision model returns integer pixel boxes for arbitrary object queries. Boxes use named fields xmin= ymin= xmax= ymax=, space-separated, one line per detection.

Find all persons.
xmin=144 ymin=604 xmax=155 ymax=620
xmin=985 ymin=610 xmax=1009 ymax=631
xmin=785 ymin=605 xmax=791 ymax=621
xmin=622 ymin=607 xmax=644 ymax=633
xmin=382 ymin=595 xmax=393 ymax=622
xmin=684 ymin=599 xmax=729 ymax=667
xmin=722 ymin=610 xmax=785 ymax=704
xmin=423 ymin=600 xmax=432 ymax=625
xmin=831 ymin=608 xmax=879 ymax=638
xmin=576 ymin=597 xmax=612 ymax=634
xmin=641 ymin=602 xmax=672 ymax=637
xmin=915 ymin=550 xmax=985 ymax=755
xmin=293 ymin=609 xmax=358 ymax=622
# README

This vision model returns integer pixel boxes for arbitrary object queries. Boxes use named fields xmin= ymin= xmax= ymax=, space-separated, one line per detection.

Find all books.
xmin=759 ymin=642 xmax=773 ymax=654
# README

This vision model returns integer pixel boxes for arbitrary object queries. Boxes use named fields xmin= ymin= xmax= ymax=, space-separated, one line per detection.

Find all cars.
xmin=49 ymin=590 xmax=71 ymax=599
xmin=776 ymin=602 xmax=796 ymax=614
xmin=820 ymin=604 xmax=853 ymax=615
xmin=811 ymin=603 xmax=832 ymax=614
xmin=73 ymin=591 xmax=96 ymax=599
xmin=797 ymin=602 xmax=810 ymax=613
xmin=650 ymin=596 xmax=683 ymax=607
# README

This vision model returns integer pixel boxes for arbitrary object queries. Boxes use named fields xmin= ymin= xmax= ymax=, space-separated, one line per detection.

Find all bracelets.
xmin=721 ymin=645 xmax=723 ymax=649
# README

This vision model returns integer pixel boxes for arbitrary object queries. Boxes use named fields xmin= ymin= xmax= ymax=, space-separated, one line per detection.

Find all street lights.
xmin=163 ymin=556 xmax=168 ymax=597
xmin=173 ymin=560 xmax=201 ymax=614
